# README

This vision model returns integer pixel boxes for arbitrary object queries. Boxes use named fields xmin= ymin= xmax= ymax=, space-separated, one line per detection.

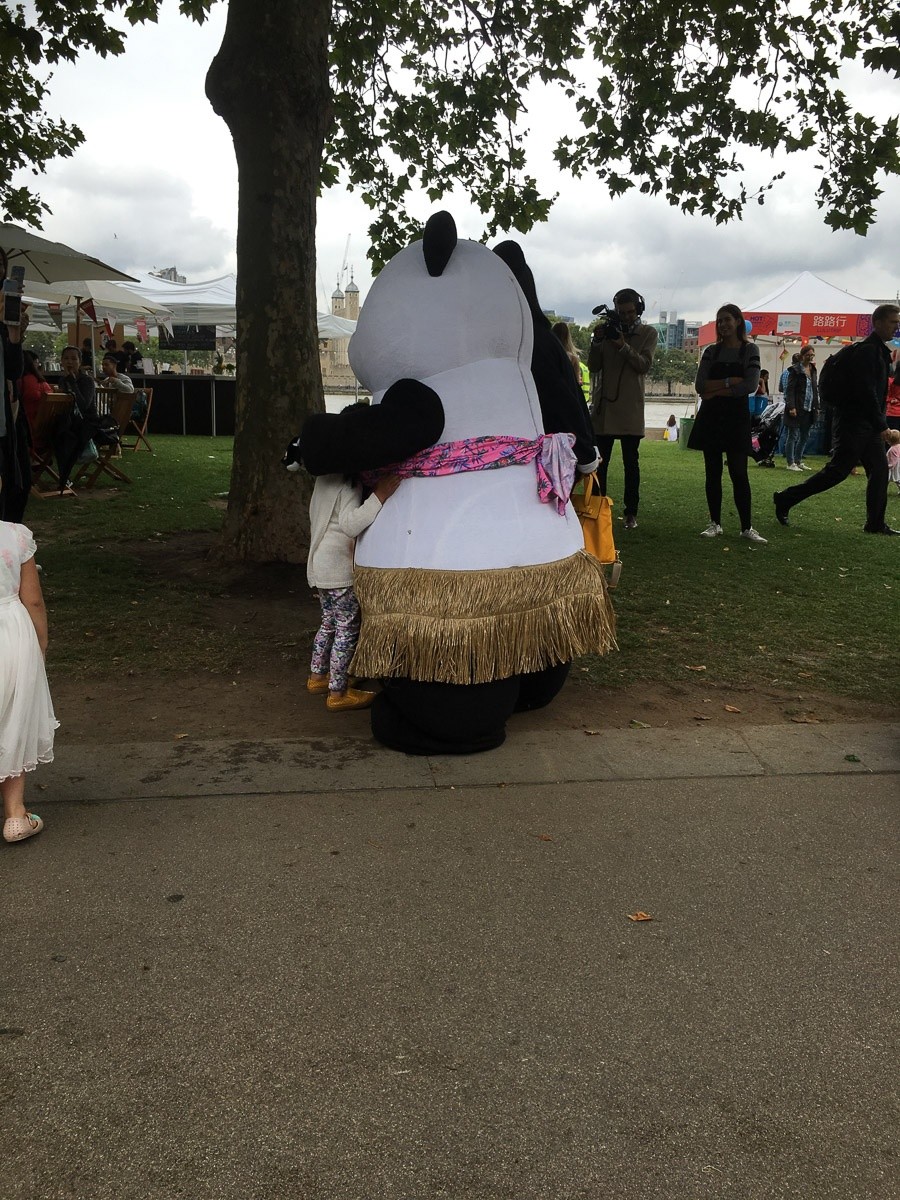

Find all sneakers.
xmin=787 ymin=463 xmax=803 ymax=471
xmin=798 ymin=463 xmax=812 ymax=470
xmin=740 ymin=526 xmax=769 ymax=544
xmin=700 ymin=521 xmax=723 ymax=537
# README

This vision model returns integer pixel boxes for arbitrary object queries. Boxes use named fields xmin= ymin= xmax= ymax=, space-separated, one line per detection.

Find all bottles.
xmin=37 ymin=361 xmax=61 ymax=371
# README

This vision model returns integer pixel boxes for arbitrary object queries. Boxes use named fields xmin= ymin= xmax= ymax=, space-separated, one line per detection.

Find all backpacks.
xmin=819 ymin=341 xmax=869 ymax=415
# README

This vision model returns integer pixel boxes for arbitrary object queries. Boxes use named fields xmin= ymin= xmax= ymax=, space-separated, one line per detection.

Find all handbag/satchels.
xmin=570 ymin=470 xmax=616 ymax=565
xmin=90 ymin=414 xmax=120 ymax=445
xmin=664 ymin=428 xmax=668 ymax=438
xmin=76 ymin=438 xmax=99 ymax=464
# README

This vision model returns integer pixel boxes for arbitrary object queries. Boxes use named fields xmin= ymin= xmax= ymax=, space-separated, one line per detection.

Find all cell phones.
xmin=4 ymin=279 xmax=19 ymax=292
xmin=2 ymin=291 xmax=21 ymax=325
xmin=10 ymin=266 xmax=25 ymax=285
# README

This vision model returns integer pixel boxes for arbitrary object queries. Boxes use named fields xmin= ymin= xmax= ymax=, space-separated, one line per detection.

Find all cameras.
xmin=601 ymin=322 xmax=624 ymax=340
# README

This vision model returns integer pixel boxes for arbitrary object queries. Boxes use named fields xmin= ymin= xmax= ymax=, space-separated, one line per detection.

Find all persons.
xmin=531 ymin=288 xmax=658 ymax=528
xmin=687 ymin=304 xmax=769 ymax=544
xmin=307 ymin=403 xmax=403 ymax=712
xmin=0 ymin=246 xmax=133 ymax=572
xmin=666 ymin=415 xmax=679 ymax=441
xmin=754 ymin=345 xmax=900 ymax=495
xmin=773 ymin=304 xmax=900 ymax=536
xmin=1 ymin=520 xmax=60 ymax=842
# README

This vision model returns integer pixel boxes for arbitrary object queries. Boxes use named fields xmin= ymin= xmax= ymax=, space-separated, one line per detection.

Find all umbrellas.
xmin=0 ymin=223 xmax=170 ymax=348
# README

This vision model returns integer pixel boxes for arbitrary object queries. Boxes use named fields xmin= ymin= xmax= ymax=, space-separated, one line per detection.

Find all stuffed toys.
xmin=284 ymin=210 xmax=620 ymax=755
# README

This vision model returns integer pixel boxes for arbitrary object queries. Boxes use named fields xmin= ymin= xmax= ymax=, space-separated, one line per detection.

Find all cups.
xmin=51 ymin=384 xmax=59 ymax=392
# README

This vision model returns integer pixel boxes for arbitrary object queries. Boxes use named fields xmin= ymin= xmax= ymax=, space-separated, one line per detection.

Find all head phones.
xmin=632 ymin=292 xmax=645 ymax=315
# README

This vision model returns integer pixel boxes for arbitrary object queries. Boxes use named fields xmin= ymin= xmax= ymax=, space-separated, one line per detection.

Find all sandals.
xmin=4 ymin=813 xmax=43 ymax=842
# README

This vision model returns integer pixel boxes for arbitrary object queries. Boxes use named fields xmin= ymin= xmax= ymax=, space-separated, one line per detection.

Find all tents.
xmin=116 ymin=274 xmax=360 ymax=403
xmin=693 ymin=272 xmax=900 ymax=420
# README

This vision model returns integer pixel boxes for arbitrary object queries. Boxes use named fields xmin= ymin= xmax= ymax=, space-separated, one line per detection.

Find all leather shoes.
xmin=773 ymin=491 xmax=790 ymax=527
xmin=863 ymin=521 xmax=900 ymax=535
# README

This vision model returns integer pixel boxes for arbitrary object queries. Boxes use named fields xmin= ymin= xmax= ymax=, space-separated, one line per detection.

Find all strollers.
xmin=747 ymin=403 xmax=792 ymax=473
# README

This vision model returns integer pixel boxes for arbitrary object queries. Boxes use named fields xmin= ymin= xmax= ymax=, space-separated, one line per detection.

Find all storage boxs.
xmin=749 ymin=396 xmax=769 ymax=417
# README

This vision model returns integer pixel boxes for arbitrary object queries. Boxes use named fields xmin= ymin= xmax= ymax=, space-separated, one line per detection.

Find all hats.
xmin=103 ymin=352 xmax=120 ymax=363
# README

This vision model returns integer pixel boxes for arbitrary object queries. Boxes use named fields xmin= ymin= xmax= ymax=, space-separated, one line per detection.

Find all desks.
xmin=45 ymin=371 xmax=236 ymax=437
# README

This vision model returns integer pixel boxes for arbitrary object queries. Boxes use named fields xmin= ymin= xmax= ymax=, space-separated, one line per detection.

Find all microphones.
xmin=592 ymin=305 xmax=605 ymax=315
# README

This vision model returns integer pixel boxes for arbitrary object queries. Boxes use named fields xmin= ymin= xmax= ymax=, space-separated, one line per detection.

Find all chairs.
xmin=27 ymin=388 xmax=153 ymax=502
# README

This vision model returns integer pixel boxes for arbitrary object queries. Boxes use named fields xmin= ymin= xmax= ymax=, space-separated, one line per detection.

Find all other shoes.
xmin=307 ymin=673 xmax=354 ymax=694
xmin=623 ymin=515 xmax=637 ymax=528
xmin=326 ymin=688 xmax=376 ymax=709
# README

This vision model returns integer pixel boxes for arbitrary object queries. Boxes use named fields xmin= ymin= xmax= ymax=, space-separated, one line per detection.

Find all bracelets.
xmin=725 ymin=378 xmax=729 ymax=388
